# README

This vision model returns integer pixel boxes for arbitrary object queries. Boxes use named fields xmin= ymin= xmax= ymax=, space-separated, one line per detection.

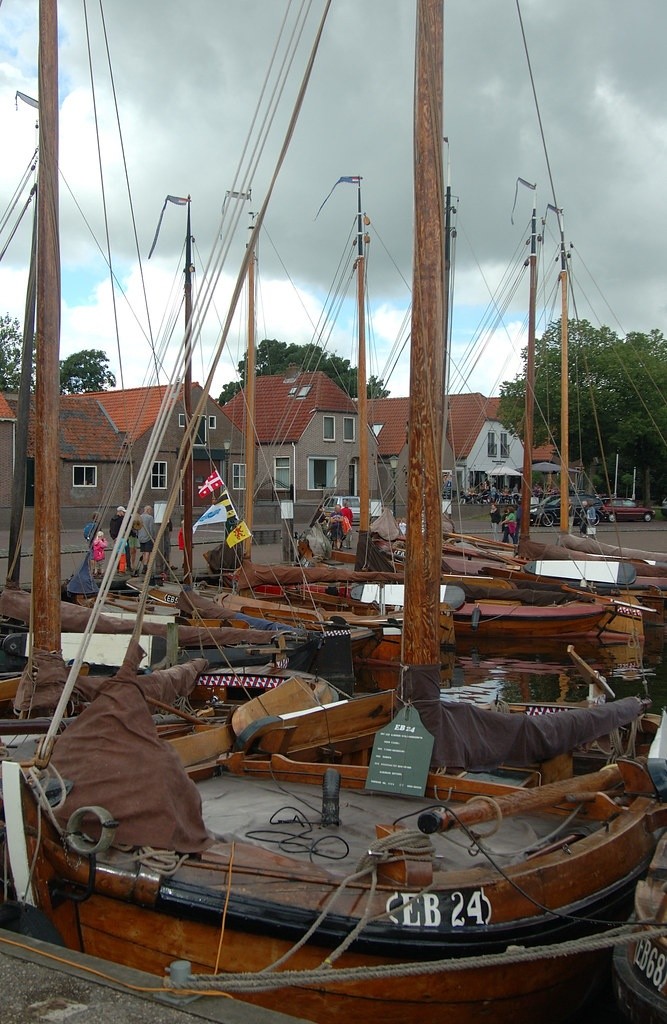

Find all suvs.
xmin=530 ymin=495 xmax=607 ymax=526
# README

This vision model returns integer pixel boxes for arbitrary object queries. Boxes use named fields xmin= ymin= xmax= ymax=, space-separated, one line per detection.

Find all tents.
xmin=485 ymin=465 xmax=523 ymax=492
xmin=514 ymin=461 xmax=582 ymax=492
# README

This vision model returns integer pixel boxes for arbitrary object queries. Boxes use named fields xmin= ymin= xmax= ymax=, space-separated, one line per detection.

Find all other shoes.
xmin=93 ymin=572 xmax=104 ymax=579
xmin=582 ymin=534 xmax=596 ymax=540
xmin=141 ymin=569 xmax=148 ymax=574
xmin=339 ymin=546 xmax=352 ymax=551
xmin=125 ymin=568 xmax=134 ymax=573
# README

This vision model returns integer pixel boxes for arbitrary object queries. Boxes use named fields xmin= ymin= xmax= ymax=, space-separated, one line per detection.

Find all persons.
xmin=156 ymin=514 xmax=178 ymax=571
xmin=109 ymin=505 xmax=160 ymax=584
xmin=329 ymin=504 xmax=344 ymax=551
xmin=341 ymin=501 xmax=354 ymax=549
xmin=580 ymin=501 xmax=588 ymax=538
xmin=489 ymin=501 xmax=502 ymax=542
xmin=178 ymin=519 xmax=185 ymax=568
xmin=92 ymin=530 xmax=108 ymax=577
xmin=491 ymin=484 xmax=497 ymax=495
xmin=511 ymin=483 xmax=523 ymax=497
xmin=533 ymin=482 xmax=542 ymax=496
xmin=502 ymin=504 xmax=522 ymax=545
xmin=586 ymin=499 xmax=597 ymax=539
xmin=545 ymin=484 xmax=553 ymax=494
xmin=83 ymin=510 xmax=102 ymax=577
xmin=568 ymin=497 xmax=574 ymax=534
xmin=309 ymin=504 xmax=328 ymax=528
xmin=502 ymin=485 xmax=509 ymax=496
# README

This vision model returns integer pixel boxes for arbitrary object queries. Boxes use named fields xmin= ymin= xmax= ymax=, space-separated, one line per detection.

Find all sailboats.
xmin=1 ymin=0 xmax=667 ymax=1024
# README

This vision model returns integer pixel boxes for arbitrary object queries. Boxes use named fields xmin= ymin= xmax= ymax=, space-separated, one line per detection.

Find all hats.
xmin=117 ymin=506 xmax=127 ymax=513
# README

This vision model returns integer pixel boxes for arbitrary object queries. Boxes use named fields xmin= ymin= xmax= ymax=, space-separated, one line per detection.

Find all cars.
xmin=661 ymin=498 xmax=667 ymax=516
xmin=542 ymin=493 xmax=554 ymax=501
xmin=600 ymin=498 xmax=655 ymax=522
xmin=324 ymin=496 xmax=375 ymax=523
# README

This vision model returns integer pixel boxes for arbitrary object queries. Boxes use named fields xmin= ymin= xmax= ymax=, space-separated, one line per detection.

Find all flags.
xmin=226 ymin=521 xmax=251 ymax=548
xmin=213 ymin=490 xmax=237 ymax=522
xmin=198 ymin=470 xmax=224 ymax=499
xmin=192 ymin=505 xmax=227 ymax=533
